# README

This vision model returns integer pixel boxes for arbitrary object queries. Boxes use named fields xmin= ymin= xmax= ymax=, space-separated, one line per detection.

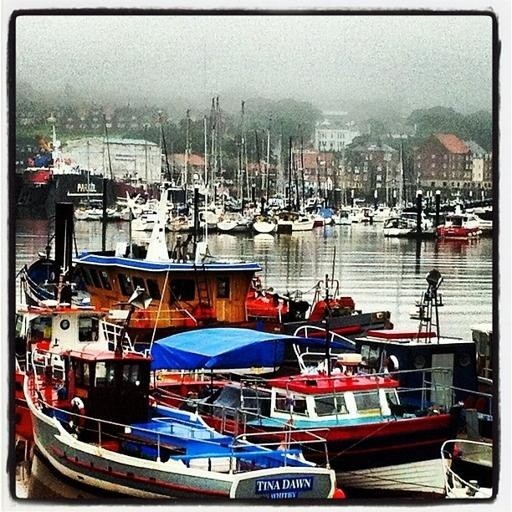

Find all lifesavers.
xmin=383 ymin=355 xmax=399 ymax=377
xmin=67 ymin=398 xmax=86 ymax=431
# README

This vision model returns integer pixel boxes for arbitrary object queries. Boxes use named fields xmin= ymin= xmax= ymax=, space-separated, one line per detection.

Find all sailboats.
xmin=14 ymin=200 xmax=83 ymax=299
xmin=72 ymin=187 xmax=263 ymax=326
xmin=151 ymin=327 xmax=493 ymax=456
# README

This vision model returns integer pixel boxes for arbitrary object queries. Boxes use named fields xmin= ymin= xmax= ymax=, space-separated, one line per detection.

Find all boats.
xmin=25 ymin=347 xmax=345 ymax=499
xmin=441 ymin=439 xmax=492 ymax=498
xmin=247 ymin=274 xmax=394 ymax=337
xmin=14 ymin=302 xmax=150 ymax=442
